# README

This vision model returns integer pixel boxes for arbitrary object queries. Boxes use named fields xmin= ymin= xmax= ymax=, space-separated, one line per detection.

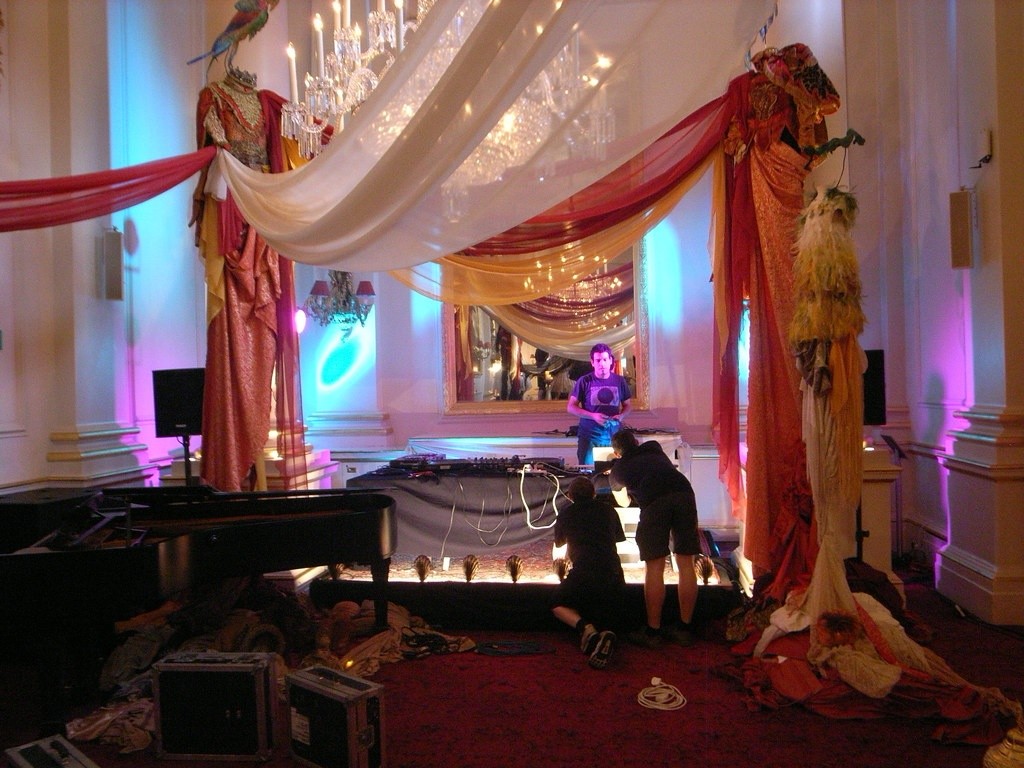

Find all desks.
xmin=346 ymin=465 xmax=644 ymax=577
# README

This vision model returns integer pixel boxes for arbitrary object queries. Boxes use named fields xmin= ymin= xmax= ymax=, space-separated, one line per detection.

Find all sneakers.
xmin=627 ymin=630 xmax=663 ymax=650
xmin=588 ymin=631 xmax=616 ymax=670
xmin=664 ymin=626 xmax=691 ymax=646
xmin=580 ymin=623 xmax=599 ymax=655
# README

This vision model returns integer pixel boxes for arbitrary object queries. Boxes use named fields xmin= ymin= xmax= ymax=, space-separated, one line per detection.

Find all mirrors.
xmin=439 ymin=235 xmax=651 ymax=414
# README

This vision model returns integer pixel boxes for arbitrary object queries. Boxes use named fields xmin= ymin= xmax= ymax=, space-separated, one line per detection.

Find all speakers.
xmin=949 ymin=192 xmax=972 ymax=269
xmin=104 ymin=231 xmax=123 ymax=299
xmin=863 ymin=351 xmax=887 ymax=426
xmin=152 ymin=368 xmax=204 ymax=437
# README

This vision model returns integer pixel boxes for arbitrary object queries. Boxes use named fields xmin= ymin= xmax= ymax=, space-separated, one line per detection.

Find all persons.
xmin=550 ymin=477 xmax=626 ymax=670
xmin=566 ymin=344 xmax=634 ymax=464
xmin=608 ymin=429 xmax=700 ymax=649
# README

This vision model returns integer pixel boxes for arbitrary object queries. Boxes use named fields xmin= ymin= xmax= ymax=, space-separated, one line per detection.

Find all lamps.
xmin=694 ymin=556 xmax=714 ymax=586
xmin=463 ymin=555 xmax=480 ymax=582
xmin=506 ymin=555 xmax=524 ymax=583
xmin=524 ymin=227 xmax=622 ymax=304
xmin=281 ymin=0 xmax=617 ymax=223
xmin=303 ymin=271 xmax=376 ymax=328
xmin=553 ymin=559 xmax=571 ymax=583
xmin=328 ymin=564 xmax=346 ymax=580
xmin=414 ymin=555 xmax=432 ymax=582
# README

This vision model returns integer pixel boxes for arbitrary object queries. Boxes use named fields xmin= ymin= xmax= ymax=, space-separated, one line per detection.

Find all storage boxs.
xmin=151 ymin=651 xmax=278 ymax=762
xmin=4 ymin=734 xmax=99 ymax=768
xmin=284 ymin=663 xmax=387 ymax=768
